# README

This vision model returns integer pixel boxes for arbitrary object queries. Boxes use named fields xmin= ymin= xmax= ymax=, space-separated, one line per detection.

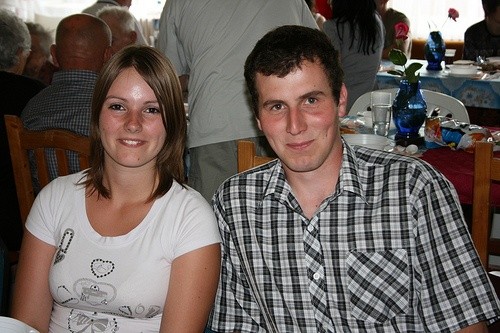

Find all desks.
xmin=422 ymin=146 xmax=500 ymax=211
xmin=375 ymin=59 xmax=500 ymax=127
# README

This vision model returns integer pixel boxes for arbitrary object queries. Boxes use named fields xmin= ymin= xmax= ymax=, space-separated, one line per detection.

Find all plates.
xmin=443 ymin=70 xmax=483 ymax=76
xmin=358 ymin=111 xmax=396 ymax=130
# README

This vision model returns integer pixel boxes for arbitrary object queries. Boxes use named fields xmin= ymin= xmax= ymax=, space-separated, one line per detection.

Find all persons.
xmin=0 ymin=0 xmax=500 ymax=208
xmin=211 ymin=25 xmax=500 ymax=333
xmin=11 ymin=44 xmax=224 ymax=333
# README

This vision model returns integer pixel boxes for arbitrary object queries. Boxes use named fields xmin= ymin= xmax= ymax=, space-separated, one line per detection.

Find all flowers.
xmin=386 ymin=22 xmax=423 ymax=121
xmin=439 ymin=8 xmax=459 ymax=32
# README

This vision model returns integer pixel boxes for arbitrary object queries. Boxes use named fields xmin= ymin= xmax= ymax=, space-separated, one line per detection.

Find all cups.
xmin=372 ymin=103 xmax=391 ymax=137
xmin=371 ymin=92 xmax=392 ymax=104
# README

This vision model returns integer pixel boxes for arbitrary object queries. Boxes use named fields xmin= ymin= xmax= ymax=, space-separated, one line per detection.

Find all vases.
xmin=392 ymin=80 xmax=427 ymax=140
xmin=424 ymin=31 xmax=446 ymax=71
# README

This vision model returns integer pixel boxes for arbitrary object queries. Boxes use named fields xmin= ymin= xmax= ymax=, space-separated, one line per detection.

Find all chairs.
xmin=470 ymin=140 xmax=500 ymax=301
xmin=4 ymin=115 xmax=94 ymax=229
xmin=348 ymin=88 xmax=471 ymax=125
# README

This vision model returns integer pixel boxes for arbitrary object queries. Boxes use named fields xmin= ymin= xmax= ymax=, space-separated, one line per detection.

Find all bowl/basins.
xmin=486 ymin=57 xmax=500 ymax=66
xmin=341 ymin=134 xmax=391 ymax=151
xmin=446 ymin=64 xmax=477 ymax=74
